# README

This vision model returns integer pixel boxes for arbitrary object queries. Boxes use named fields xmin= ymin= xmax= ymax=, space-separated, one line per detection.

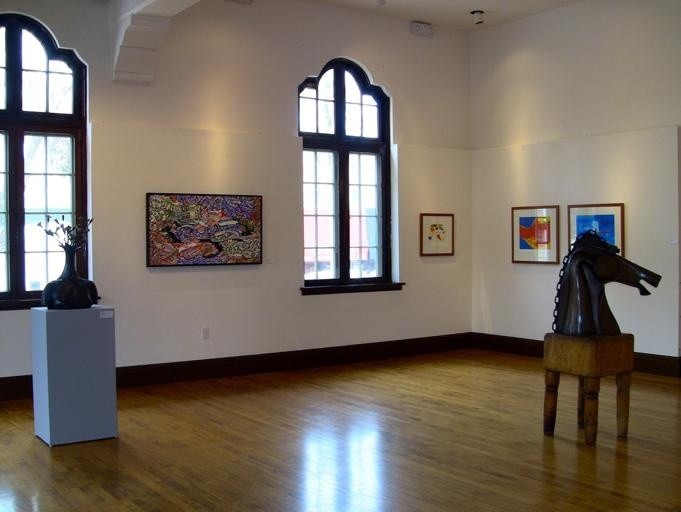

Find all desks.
xmin=539 ymin=332 xmax=636 ymax=448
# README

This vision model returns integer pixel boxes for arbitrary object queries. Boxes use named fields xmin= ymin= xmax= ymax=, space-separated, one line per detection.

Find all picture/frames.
xmin=419 ymin=212 xmax=455 ymax=257
xmin=510 ymin=205 xmax=560 ymax=266
xmin=145 ymin=191 xmax=263 ymax=269
xmin=566 ymin=202 xmax=625 ymax=260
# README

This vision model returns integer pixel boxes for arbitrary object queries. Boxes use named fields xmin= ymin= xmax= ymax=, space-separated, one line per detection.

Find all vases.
xmin=39 ymin=246 xmax=99 ymax=310
